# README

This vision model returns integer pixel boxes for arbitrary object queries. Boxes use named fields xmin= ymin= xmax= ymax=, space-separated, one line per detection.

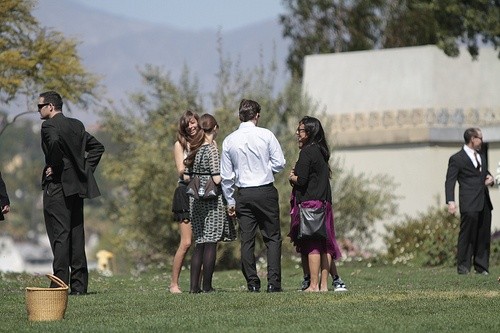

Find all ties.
xmin=474 ymin=152 xmax=480 ymax=170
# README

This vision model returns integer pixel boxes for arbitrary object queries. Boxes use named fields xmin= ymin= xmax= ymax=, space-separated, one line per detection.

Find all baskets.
xmin=25 ymin=273 xmax=68 ymax=321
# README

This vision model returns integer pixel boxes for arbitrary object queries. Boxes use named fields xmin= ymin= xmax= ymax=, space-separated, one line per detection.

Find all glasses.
xmin=258 ymin=115 xmax=260 ymax=117
xmin=298 ymin=128 xmax=305 ymax=132
xmin=475 ymin=136 xmax=483 ymax=140
xmin=38 ymin=103 xmax=54 ymax=109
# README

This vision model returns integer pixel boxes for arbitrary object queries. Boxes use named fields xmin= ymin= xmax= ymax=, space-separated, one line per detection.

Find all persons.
xmin=287 ymin=115 xmax=349 ymax=293
xmin=220 ymin=98 xmax=286 ymax=293
xmin=169 ymin=110 xmax=237 ymax=294
xmin=37 ymin=91 xmax=105 ymax=296
xmin=445 ymin=128 xmax=495 ymax=275
xmin=0 ymin=169 xmax=10 ymax=221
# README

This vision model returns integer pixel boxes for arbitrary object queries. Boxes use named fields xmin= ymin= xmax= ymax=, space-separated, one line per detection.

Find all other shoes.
xmin=333 ymin=275 xmax=348 ymax=291
xmin=249 ymin=286 xmax=260 ymax=292
xmin=475 ymin=270 xmax=488 ymax=275
xmin=267 ymin=284 xmax=284 ymax=293
xmin=301 ymin=274 xmax=310 ymax=291
